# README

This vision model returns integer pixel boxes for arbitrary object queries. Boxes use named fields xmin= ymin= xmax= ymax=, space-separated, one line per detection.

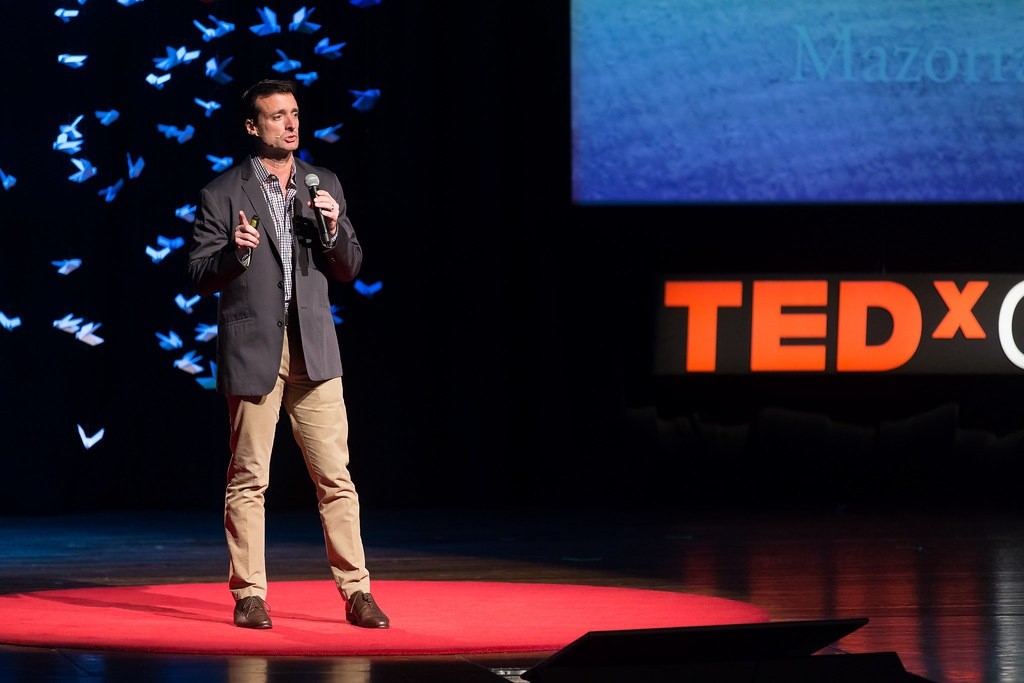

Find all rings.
xmin=329 ymin=204 xmax=336 ymax=211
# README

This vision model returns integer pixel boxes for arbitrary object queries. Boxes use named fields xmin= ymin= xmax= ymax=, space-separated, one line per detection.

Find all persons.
xmin=185 ymin=78 xmax=391 ymax=631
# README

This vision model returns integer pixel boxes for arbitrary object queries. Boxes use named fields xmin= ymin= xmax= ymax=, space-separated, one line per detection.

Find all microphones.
xmin=304 ymin=173 xmax=331 ymax=245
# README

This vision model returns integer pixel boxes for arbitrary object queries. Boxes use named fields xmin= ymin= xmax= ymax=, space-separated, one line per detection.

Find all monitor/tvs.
xmin=520 ymin=616 xmax=871 ymax=682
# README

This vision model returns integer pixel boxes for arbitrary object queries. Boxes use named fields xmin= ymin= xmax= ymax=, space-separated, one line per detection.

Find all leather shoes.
xmin=345 ymin=590 xmax=389 ymax=628
xmin=234 ymin=596 xmax=273 ymax=628
